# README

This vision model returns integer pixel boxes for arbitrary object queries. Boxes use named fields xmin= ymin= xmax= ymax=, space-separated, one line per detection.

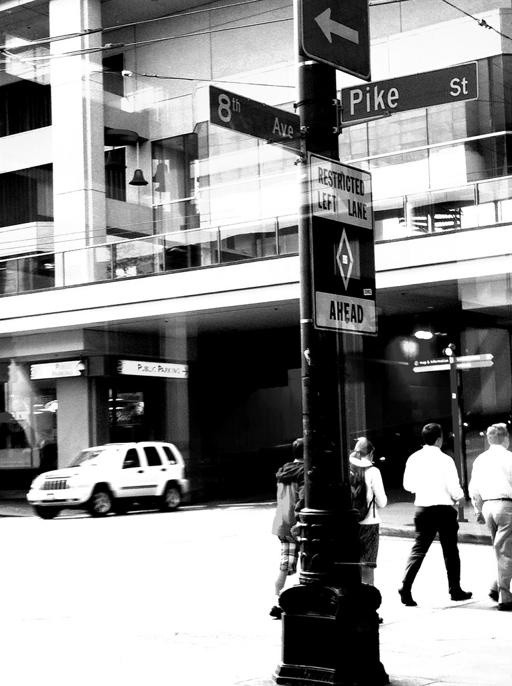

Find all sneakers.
xmin=490 ymin=590 xmax=511 ymax=611
xmin=399 ymin=586 xmax=417 ymax=606
xmin=270 ymin=606 xmax=283 ymax=618
xmin=451 ymin=592 xmax=472 ymax=601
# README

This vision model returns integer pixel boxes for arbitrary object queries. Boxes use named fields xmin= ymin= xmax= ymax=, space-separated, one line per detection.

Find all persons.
xmin=467 ymin=422 xmax=512 ymax=612
xmin=397 ymin=422 xmax=473 ymax=608
xmin=346 ymin=436 xmax=388 ymax=624
xmin=268 ymin=437 xmax=305 ymax=620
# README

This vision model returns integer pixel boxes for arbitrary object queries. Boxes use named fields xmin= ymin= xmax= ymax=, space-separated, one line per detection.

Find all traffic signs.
xmin=340 ymin=61 xmax=479 ymax=127
xmin=208 ymin=85 xmax=302 ymax=153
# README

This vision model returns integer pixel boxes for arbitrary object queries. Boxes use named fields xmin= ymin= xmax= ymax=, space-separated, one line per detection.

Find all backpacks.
xmin=349 ymin=462 xmax=375 ymax=522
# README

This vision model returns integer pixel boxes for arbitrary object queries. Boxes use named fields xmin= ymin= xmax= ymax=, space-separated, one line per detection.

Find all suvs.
xmin=26 ymin=439 xmax=190 ymax=519
